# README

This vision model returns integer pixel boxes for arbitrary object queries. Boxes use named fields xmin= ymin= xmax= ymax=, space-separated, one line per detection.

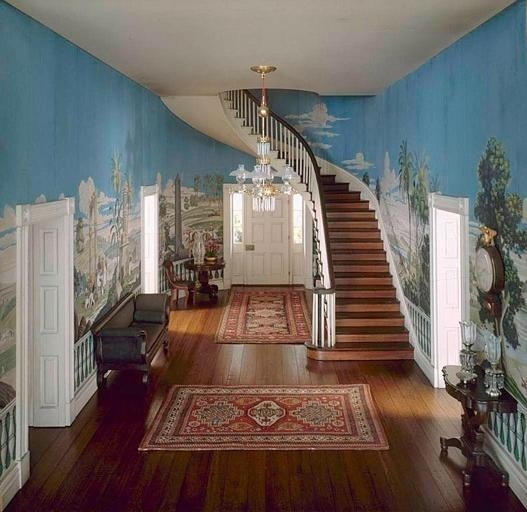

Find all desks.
xmin=184 ymin=259 xmax=226 ymax=306
xmin=440 ymin=364 xmax=517 ymax=498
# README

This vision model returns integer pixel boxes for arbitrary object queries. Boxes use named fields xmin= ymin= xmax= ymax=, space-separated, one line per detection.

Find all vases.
xmin=204 ymin=256 xmax=216 ymax=261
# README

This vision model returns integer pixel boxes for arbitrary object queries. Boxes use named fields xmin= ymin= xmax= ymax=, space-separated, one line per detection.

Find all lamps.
xmin=229 ymin=66 xmax=293 ymax=202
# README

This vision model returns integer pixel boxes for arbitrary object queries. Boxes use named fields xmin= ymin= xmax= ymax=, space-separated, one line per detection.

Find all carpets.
xmin=214 ymin=286 xmax=312 ymax=344
xmin=137 ymin=383 xmax=390 ymax=451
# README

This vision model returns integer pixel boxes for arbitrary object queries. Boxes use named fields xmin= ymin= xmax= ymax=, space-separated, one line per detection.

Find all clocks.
xmin=474 ymin=226 xmax=504 ymax=324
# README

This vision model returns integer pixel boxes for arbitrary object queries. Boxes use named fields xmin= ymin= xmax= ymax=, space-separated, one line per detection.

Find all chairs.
xmin=164 ymin=261 xmax=195 ymax=308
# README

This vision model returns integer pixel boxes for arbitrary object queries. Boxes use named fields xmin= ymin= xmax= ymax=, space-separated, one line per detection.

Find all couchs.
xmin=90 ymin=292 xmax=171 ymax=394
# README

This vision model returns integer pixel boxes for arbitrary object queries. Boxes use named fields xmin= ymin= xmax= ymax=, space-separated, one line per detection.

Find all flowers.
xmin=205 ymin=240 xmax=219 ymax=257
